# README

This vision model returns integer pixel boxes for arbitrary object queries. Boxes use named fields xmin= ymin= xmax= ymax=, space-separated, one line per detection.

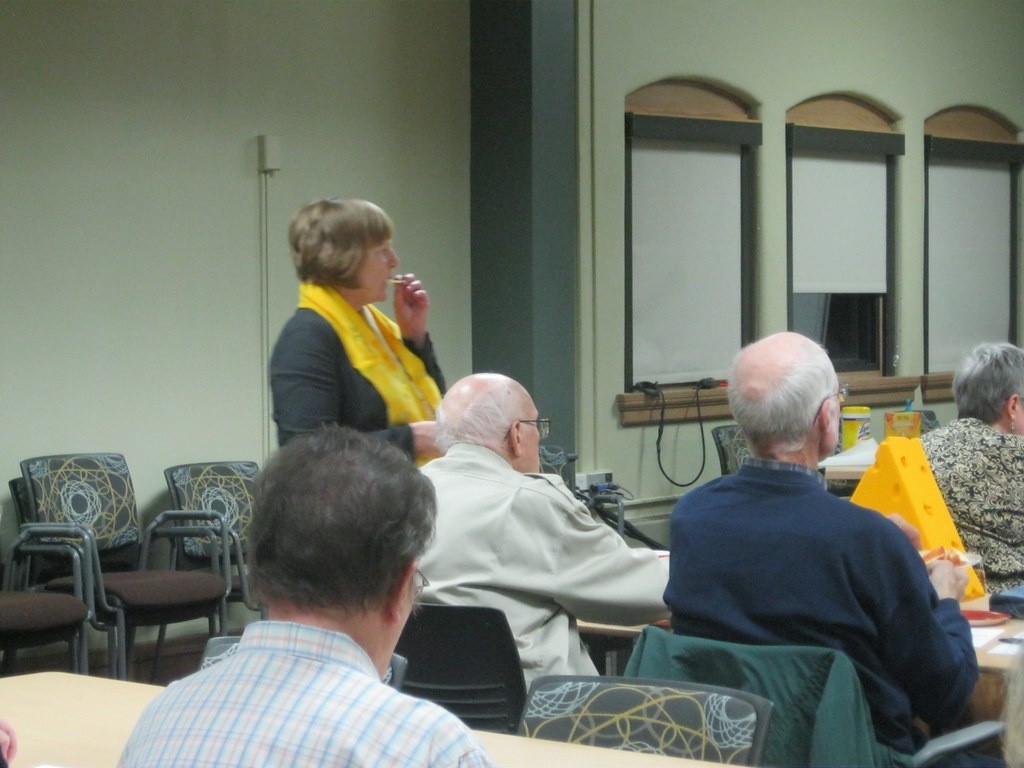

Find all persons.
xmin=662 ymin=332 xmax=1024 ymax=768
xmin=414 ymin=372 xmax=669 ymax=693
xmin=117 ymin=423 xmax=491 ymax=768
xmin=270 ymin=199 xmax=447 ymax=468
xmin=0 ymin=722 xmax=17 ymax=768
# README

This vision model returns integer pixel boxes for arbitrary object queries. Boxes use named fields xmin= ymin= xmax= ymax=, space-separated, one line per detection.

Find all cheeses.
xmin=848 ymin=436 xmax=985 ymax=605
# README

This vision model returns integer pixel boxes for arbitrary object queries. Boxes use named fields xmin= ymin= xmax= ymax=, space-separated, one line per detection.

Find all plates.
xmin=961 ymin=610 xmax=1012 ymax=625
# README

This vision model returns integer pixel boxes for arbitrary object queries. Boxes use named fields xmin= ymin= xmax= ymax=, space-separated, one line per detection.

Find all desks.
xmin=0 ymin=611 xmax=1024 ymax=768
xmin=817 ymin=438 xmax=880 ymax=494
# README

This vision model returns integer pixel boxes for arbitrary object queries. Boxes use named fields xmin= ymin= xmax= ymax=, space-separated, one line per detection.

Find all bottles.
xmin=841 ymin=406 xmax=871 ymax=451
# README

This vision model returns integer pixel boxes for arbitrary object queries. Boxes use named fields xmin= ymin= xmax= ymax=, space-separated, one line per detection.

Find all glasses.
xmin=414 ymin=568 xmax=429 ymax=598
xmin=812 ymin=383 xmax=849 ymax=425
xmin=503 ymin=419 xmax=550 ymax=440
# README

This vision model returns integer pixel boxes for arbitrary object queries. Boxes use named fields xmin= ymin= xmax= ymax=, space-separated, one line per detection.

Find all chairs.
xmin=514 ymin=675 xmax=772 ymax=768
xmin=711 ymin=425 xmax=752 ymax=476
xmin=625 ymin=626 xmax=1007 ymax=768
xmin=0 ymin=451 xmax=271 ymax=681
xmin=396 ymin=605 xmax=527 ymax=733
xmin=201 ymin=634 xmax=407 ymax=694
